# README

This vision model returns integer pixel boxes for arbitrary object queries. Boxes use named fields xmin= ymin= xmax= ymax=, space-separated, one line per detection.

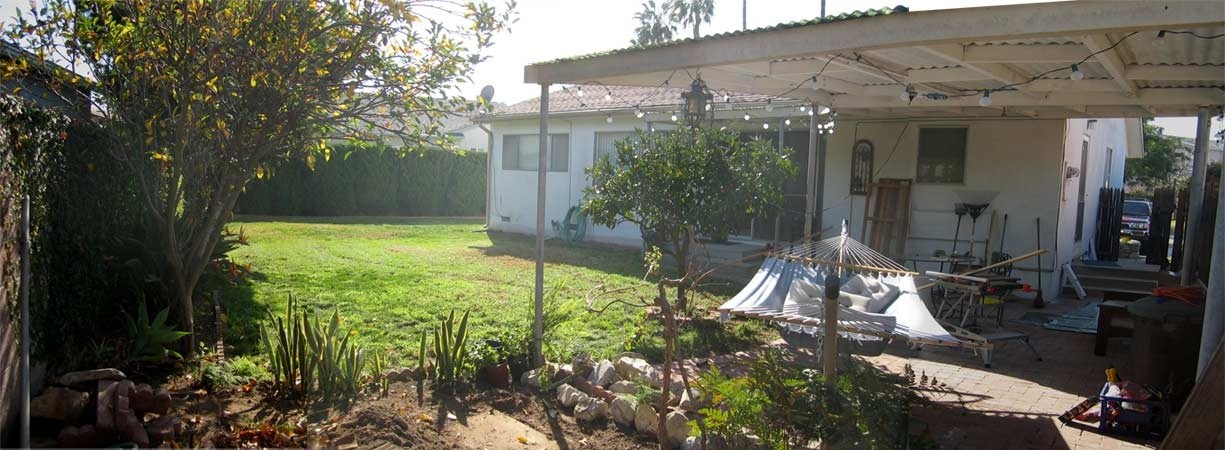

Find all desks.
xmin=1093 ymin=298 xmax=1140 ymax=356
xmin=903 ymin=255 xmax=1024 ymax=326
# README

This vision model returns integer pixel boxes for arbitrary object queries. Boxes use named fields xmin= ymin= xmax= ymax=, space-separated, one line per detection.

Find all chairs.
xmin=980 ymin=253 xmax=1015 ymax=320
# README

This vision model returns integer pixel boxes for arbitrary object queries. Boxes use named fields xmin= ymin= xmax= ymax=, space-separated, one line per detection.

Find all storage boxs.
xmin=1101 ymin=380 xmax=1171 ymax=439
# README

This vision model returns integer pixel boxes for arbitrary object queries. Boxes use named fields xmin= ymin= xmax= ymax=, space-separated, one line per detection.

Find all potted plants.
xmin=434 ymin=274 xmax=582 ymax=394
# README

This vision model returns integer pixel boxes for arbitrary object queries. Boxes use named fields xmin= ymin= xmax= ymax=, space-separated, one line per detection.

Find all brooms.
xmin=1032 ymin=217 xmax=1045 ymax=307
xmin=948 ymin=202 xmax=969 ymax=273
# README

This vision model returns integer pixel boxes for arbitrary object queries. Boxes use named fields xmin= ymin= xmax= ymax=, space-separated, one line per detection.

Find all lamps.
xmin=681 ymin=68 xmax=714 ymax=121
xmin=947 ymin=188 xmax=999 ymax=271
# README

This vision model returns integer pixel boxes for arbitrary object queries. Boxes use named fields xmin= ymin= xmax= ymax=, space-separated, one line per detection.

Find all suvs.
xmin=1119 ymin=199 xmax=1154 ymax=245
